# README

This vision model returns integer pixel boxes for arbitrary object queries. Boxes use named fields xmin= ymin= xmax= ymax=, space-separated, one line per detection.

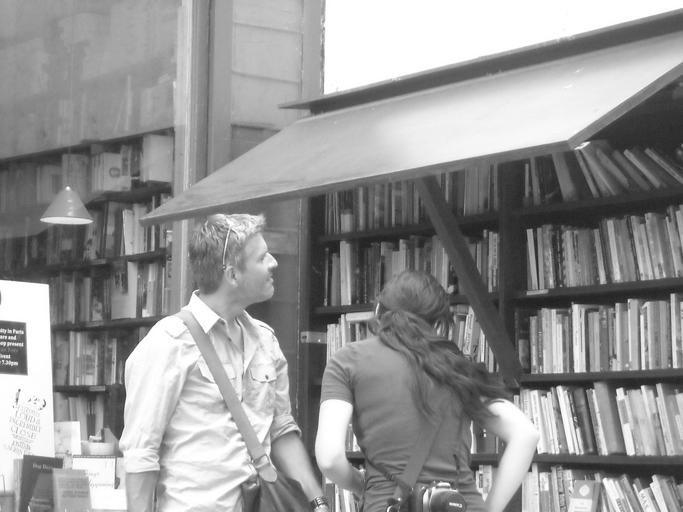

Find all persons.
xmin=118 ymin=213 xmax=330 ymax=512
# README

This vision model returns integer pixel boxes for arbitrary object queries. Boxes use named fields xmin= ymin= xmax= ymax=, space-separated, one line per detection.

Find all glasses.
xmin=222 ymin=217 xmax=238 ymax=271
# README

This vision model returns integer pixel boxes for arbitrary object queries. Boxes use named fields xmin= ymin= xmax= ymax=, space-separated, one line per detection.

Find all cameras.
xmin=408 ymin=481 xmax=467 ymax=512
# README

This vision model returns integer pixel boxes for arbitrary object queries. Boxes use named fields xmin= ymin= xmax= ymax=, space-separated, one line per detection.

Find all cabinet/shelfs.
xmin=311 ymin=162 xmax=683 ymax=512
xmin=0 ymin=181 xmax=172 ymax=393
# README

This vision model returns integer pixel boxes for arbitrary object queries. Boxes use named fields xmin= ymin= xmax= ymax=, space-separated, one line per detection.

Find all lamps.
xmin=40 ymin=0 xmax=95 ymax=225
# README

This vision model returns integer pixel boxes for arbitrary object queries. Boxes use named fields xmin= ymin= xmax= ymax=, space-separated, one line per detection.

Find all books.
xmin=474 ymin=463 xmax=683 ymax=512
xmin=54 ymin=385 xmax=125 ymax=449
xmin=1 ymin=131 xmax=173 ymax=270
xmin=324 ymin=310 xmax=385 ymax=509
xmin=45 ymin=260 xmax=172 ymax=384
xmin=434 ymin=297 xmax=683 ymax=456
xmin=0 ymin=454 xmax=92 ymax=512
xmin=317 ymin=135 xmax=683 ymax=305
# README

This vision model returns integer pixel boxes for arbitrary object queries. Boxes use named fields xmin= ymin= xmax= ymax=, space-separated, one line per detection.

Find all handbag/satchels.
xmin=240 ymin=474 xmax=313 ymax=512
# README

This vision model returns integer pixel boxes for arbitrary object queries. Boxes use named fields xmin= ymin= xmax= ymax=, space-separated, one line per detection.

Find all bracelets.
xmin=310 ymin=495 xmax=330 ymax=511
